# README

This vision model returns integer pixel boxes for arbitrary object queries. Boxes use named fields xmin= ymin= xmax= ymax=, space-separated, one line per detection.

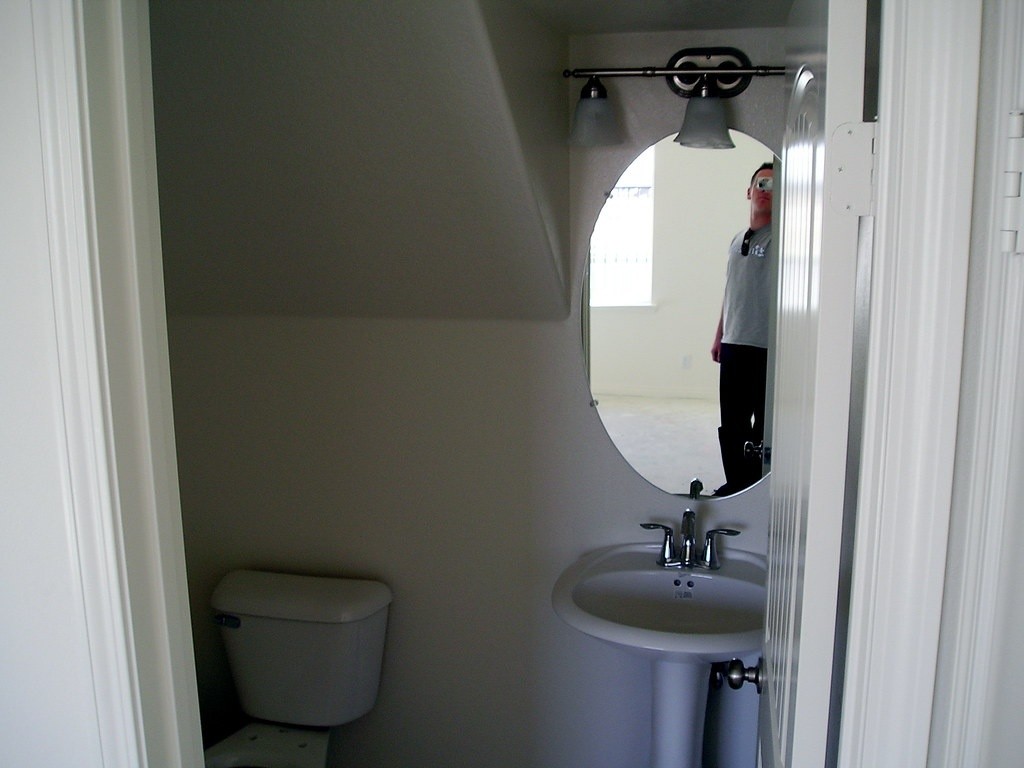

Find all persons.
xmin=711 ymin=162 xmax=772 ymax=498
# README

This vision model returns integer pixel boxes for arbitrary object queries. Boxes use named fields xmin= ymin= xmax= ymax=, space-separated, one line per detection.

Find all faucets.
xmin=689 ymin=477 xmax=704 ymax=500
xmin=680 ymin=508 xmax=697 ymax=571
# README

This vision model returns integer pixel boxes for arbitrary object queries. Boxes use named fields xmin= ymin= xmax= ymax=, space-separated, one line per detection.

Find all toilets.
xmin=203 ymin=568 xmax=395 ymax=768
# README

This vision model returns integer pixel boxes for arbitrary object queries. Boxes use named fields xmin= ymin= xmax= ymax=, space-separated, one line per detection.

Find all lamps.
xmin=673 ymin=75 xmax=735 ymax=150
xmin=566 ymin=77 xmax=623 ymax=146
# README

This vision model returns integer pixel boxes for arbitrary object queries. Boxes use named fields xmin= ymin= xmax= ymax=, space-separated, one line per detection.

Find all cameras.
xmin=756 ymin=177 xmax=773 ymax=191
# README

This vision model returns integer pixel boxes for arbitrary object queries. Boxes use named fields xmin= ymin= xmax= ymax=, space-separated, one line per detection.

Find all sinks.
xmin=550 ymin=540 xmax=770 ymax=664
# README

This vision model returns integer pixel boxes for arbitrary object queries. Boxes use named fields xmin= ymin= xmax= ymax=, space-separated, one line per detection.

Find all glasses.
xmin=751 ymin=176 xmax=774 ymax=191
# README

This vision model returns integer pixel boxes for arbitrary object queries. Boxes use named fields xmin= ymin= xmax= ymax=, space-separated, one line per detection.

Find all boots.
xmin=713 ymin=427 xmax=763 ymax=497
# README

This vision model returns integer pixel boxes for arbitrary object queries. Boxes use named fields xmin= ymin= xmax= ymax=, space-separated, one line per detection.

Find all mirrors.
xmin=580 ymin=129 xmax=784 ymax=500
